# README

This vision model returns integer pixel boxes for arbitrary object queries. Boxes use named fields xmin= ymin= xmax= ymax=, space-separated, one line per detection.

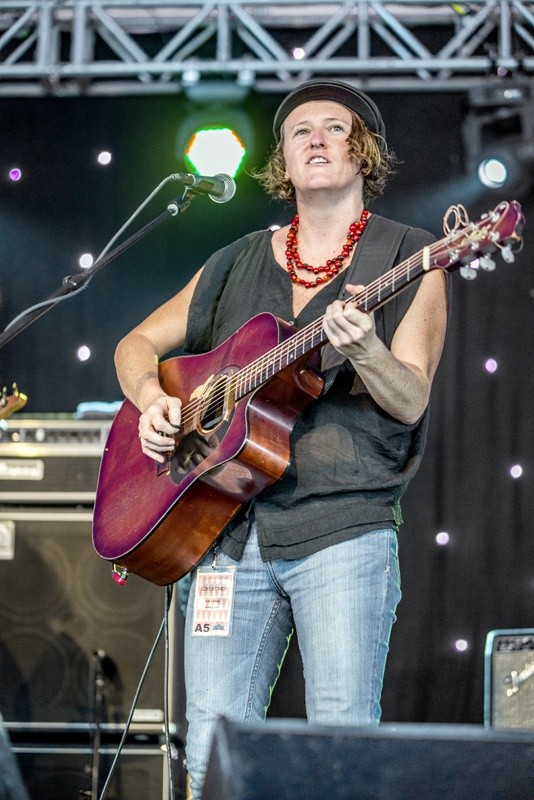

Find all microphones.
xmin=170 ymin=172 xmax=238 ymax=204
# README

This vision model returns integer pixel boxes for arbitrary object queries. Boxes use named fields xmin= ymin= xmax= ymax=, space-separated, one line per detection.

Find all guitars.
xmin=0 ymin=382 xmax=28 ymax=419
xmin=91 ymin=200 xmax=525 ymax=588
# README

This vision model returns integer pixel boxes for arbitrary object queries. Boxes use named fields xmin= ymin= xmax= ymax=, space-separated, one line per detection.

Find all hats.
xmin=273 ymin=80 xmax=386 ymax=149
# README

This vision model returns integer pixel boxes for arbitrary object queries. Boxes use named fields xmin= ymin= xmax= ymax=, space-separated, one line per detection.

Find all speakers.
xmin=10 ymin=745 xmax=183 ymax=800
xmin=0 ymin=510 xmax=181 ymax=737
xmin=483 ymin=628 xmax=534 ymax=736
xmin=200 ymin=714 xmax=534 ymax=800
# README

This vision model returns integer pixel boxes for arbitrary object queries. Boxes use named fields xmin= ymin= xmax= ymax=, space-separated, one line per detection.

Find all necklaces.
xmin=285 ymin=207 xmax=368 ymax=289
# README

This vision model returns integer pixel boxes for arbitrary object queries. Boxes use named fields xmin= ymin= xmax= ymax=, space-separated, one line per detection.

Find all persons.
xmin=113 ymin=80 xmax=454 ymax=800
